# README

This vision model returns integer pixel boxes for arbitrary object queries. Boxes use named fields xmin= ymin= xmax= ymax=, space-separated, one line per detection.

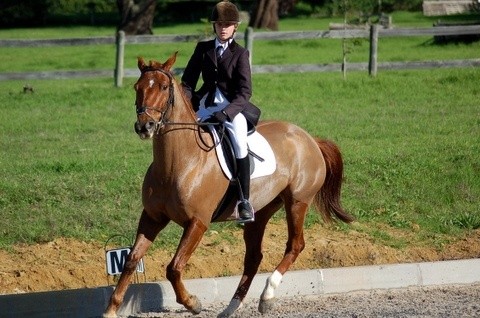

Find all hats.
xmin=210 ymin=2 xmax=240 ymax=23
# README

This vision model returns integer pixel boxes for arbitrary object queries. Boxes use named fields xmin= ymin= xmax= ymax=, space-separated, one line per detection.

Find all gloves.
xmin=210 ymin=111 xmax=227 ymax=125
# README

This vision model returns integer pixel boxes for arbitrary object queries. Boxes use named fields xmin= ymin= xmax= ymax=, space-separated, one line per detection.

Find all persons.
xmin=181 ymin=0 xmax=262 ymax=220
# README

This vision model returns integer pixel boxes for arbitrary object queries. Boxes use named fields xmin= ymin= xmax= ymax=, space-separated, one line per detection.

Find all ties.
xmin=204 ymin=45 xmax=224 ymax=109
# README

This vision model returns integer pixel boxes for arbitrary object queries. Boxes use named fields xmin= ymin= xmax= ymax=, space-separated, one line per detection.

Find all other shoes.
xmin=238 ymin=202 xmax=253 ymax=219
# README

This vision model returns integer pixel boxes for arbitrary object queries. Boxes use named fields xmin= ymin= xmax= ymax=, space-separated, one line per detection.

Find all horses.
xmin=102 ymin=50 xmax=356 ymax=318
